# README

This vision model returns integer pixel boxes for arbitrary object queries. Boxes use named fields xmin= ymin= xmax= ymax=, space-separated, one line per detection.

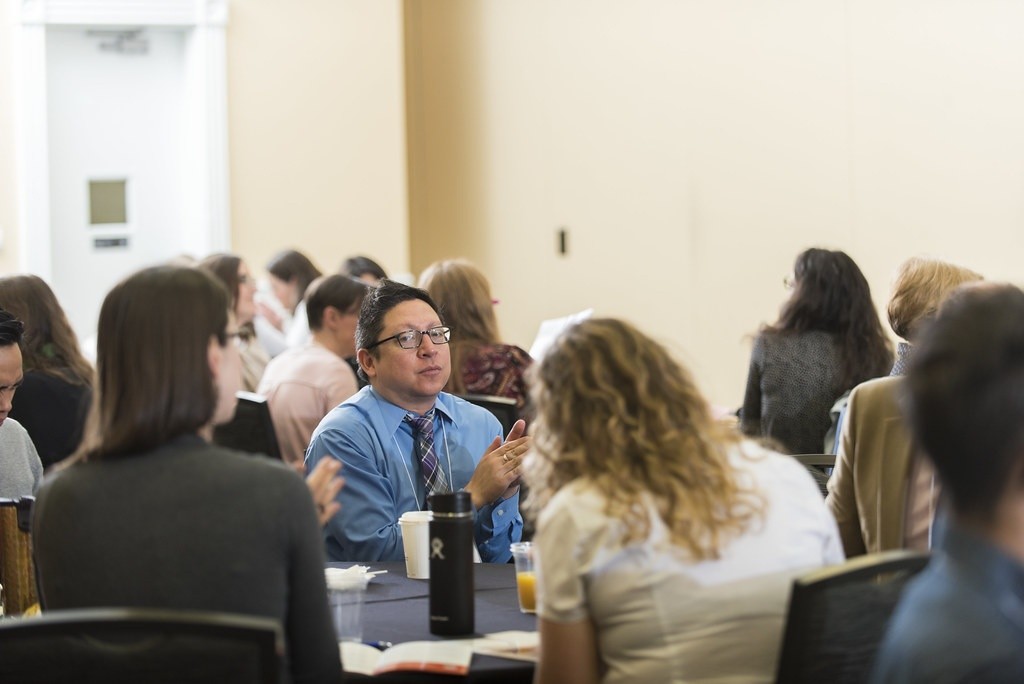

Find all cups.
xmin=398 ymin=511 xmax=433 ymax=579
xmin=509 ymin=540 xmax=536 ymax=613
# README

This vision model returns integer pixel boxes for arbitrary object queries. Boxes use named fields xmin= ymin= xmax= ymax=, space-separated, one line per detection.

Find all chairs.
xmin=213 ymin=392 xmax=281 ymax=462
xmin=786 ymin=454 xmax=836 ymax=499
xmin=0 ymin=608 xmax=286 ymax=684
xmin=454 ymin=393 xmax=518 ymax=441
xmin=775 ymin=551 xmax=930 ymax=682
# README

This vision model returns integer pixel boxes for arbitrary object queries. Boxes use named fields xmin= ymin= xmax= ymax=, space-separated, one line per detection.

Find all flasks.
xmin=427 ymin=492 xmax=476 ymax=638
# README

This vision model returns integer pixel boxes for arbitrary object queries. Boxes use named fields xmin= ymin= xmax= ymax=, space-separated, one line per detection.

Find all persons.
xmin=421 ymin=260 xmax=535 ymax=399
xmin=887 ymin=259 xmax=982 ymax=377
xmin=871 ymin=283 xmax=1024 ymax=684
xmin=304 ymin=279 xmax=532 ymax=565
xmin=29 ymin=266 xmax=347 ymax=684
xmin=826 ymin=378 xmax=943 ymax=556
xmin=741 ymin=250 xmax=894 ymax=472
xmin=529 ymin=317 xmax=843 ymax=684
xmin=1 ymin=275 xmax=94 ymax=497
xmin=202 ymin=250 xmax=389 ymax=465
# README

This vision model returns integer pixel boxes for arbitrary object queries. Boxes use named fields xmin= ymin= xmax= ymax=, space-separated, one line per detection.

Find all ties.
xmin=403 ymin=410 xmax=447 ymax=498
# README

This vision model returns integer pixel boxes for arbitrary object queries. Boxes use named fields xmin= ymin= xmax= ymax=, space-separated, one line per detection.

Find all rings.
xmin=504 ymin=453 xmax=508 ymax=461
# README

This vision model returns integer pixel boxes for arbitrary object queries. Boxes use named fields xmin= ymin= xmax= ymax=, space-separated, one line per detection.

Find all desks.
xmin=324 ymin=559 xmax=538 ymax=684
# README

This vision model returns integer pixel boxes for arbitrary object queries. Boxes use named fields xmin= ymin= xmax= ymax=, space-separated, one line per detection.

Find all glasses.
xmin=781 ymin=277 xmax=794 ymax=285
xmin=223 ymin=324 xmax=251 ymax=352
xmin=365 ymin=326 xmax=451 ymax=349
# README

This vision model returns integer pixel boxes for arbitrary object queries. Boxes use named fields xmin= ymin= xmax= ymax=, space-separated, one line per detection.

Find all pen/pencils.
xmin=354 ymin=639 xmax=391 ymax=649
